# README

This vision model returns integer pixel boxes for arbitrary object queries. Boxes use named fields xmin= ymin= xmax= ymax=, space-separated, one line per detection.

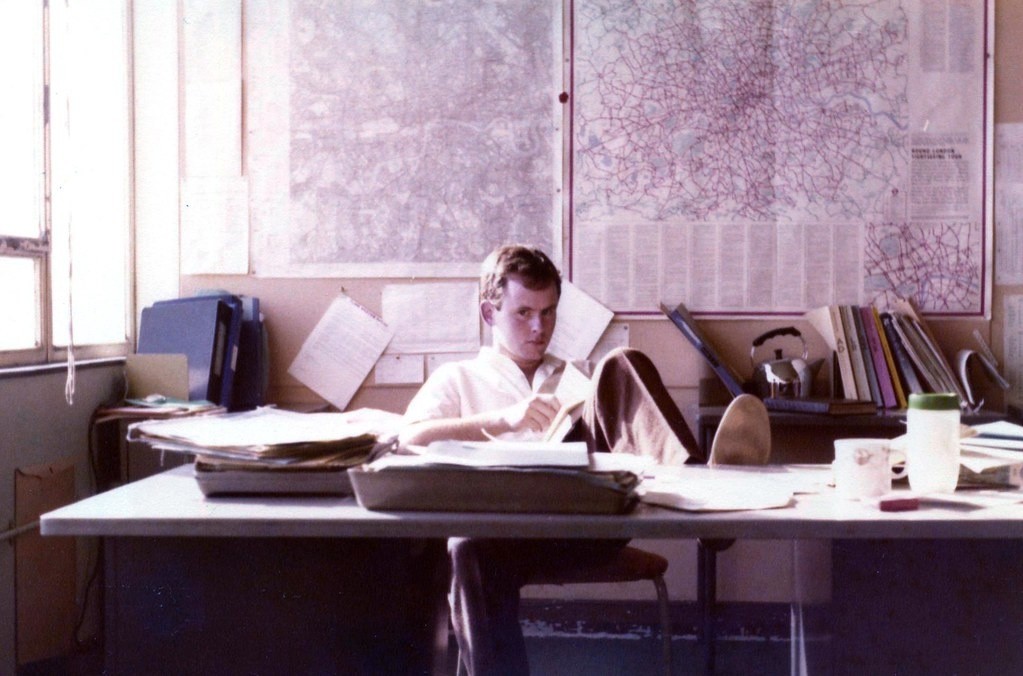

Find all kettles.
xmin=747 ymin=324 xmax=827 ymax=400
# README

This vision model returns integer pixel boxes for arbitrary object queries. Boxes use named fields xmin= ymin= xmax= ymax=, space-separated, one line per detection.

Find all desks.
xmin=696 ymin=404 xmax=1022 ymax=676
xmin=40 ymin=465 xmax=1022 ymax=675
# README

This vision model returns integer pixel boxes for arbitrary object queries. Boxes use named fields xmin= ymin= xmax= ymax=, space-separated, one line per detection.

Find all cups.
xmin=905 ymin=392 xmax=966 ymax=494
xmin=834 ymin=437 xmax=892 ymax=500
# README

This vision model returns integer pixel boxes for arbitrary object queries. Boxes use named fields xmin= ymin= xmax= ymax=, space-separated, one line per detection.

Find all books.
xmin=371 ymin=420 xmax=647 ymax=476
xmin=125 ymin=401 xmax=388 ymax=468
xmin=807 ymin=296 xmax=1011 ymax=408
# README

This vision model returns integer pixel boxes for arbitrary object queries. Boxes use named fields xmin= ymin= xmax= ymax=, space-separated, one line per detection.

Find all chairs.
xmin=455 ymin=544 xmax=672 ymax=675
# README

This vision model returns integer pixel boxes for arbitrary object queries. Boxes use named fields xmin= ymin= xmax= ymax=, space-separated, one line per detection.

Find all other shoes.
xmin=693 ymin=393 xmax=771 ymax=553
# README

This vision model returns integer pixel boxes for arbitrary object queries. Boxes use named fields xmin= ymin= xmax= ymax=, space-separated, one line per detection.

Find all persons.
xmin=395 ymin=242 xmax=773 ymax=676
